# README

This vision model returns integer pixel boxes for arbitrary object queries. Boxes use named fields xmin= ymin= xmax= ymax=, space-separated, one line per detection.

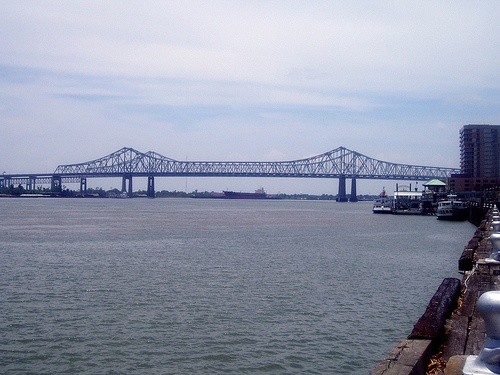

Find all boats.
xmin=373 ymin=186 xmax=392 ymax=212
xmin=437 ymin=195 xmax=469 ymax=220
